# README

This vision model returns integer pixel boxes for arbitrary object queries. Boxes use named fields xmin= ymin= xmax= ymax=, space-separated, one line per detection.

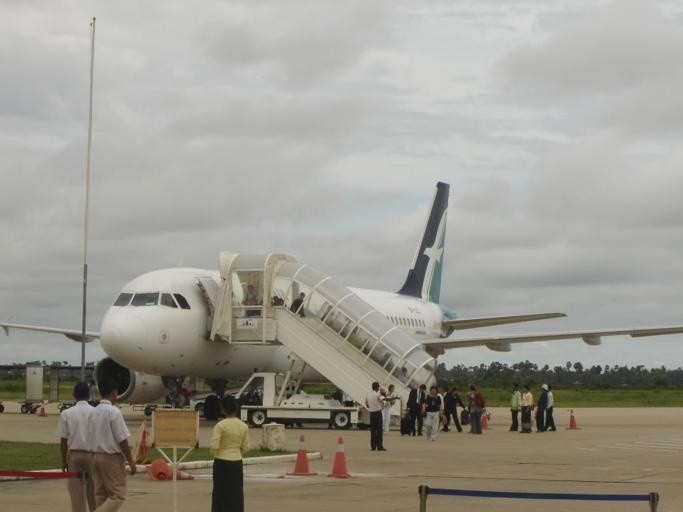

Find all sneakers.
xmin=509 ymin=427 xmax=557 ymax=433
xmin=426 ymin=434 xmax=437 ymax=441
xmin=443 ymin=426 xmax=482 ymax=435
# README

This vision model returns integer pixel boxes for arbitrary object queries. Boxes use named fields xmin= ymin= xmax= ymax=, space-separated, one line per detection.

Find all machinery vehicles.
xmin=203 ymin=249 xmax=438 ymax=430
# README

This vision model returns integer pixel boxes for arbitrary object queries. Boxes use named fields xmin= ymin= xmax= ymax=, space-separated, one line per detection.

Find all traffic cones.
xmin=285 ymin=433 xmax=319 ymax=475
xmin=36 ymin=399 xmax=47 ymax=417
xmin=148 ymin=459 xmax=192 ymax=480
xmin=565 ymin=409 xmax=581 ymax=430
xmin=326 ymin=434 xmax=350 ymax=478
xmin=480 ymin=408 xmax=492 ymax=431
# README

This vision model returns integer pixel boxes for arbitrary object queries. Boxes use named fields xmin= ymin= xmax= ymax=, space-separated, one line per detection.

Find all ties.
xmin=418 ymin=392 xmax=421 ymax=404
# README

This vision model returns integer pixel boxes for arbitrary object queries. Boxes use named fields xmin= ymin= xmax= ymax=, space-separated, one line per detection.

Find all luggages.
xmin=399 ymin=416 xmax=414 ymax=437
xmin=461 ymin=409 xmax=469 ymax=426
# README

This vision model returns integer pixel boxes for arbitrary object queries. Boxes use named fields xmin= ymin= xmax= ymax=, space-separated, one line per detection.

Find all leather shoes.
xmin=418 ymin=432 xmax=423 ymax=436
xmin=377 ymin=448 xmax=388 ymax=451
xmin=371 ymin=447 xmax=377 ymax=450
xmin=412 ymin=433 xmax=417 ymax=437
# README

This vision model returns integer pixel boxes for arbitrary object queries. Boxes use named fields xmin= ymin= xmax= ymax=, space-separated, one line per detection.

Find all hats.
xmin=541 ymin=384 xmax=549 ymax=391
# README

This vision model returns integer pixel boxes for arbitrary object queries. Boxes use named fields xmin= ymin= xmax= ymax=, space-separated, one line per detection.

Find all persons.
xmin=536 ymin=384 xmax=549 ymax=432
xmin=544 ymin=385 xmax=556 ymax=431
xmin=520 ymin=385 xmax=534 ymax=433
xmin=210 ymin=397 xmax=250 ymax=512
xmin=88 ymin=380 xmax=95 ymax=401
xmin=508 ymin=383 xmax=521 ymax=431
xmin=88 ymin=379 xmax=136 ymax=512
xmin=58 ymin=383 xmax=95 ymax=512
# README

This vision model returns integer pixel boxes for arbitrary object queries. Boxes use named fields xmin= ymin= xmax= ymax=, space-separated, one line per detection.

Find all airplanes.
xmin=0 ymin=180 xmax=681 ymax=418
